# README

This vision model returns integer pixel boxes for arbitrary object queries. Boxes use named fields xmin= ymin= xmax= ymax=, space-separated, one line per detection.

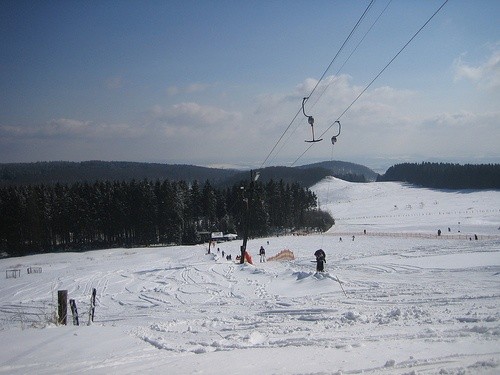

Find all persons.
xmin=259 ymin=246 xmax=266 ymax=263
xmin=313 ymin=248 xmax=326 ymax=272
